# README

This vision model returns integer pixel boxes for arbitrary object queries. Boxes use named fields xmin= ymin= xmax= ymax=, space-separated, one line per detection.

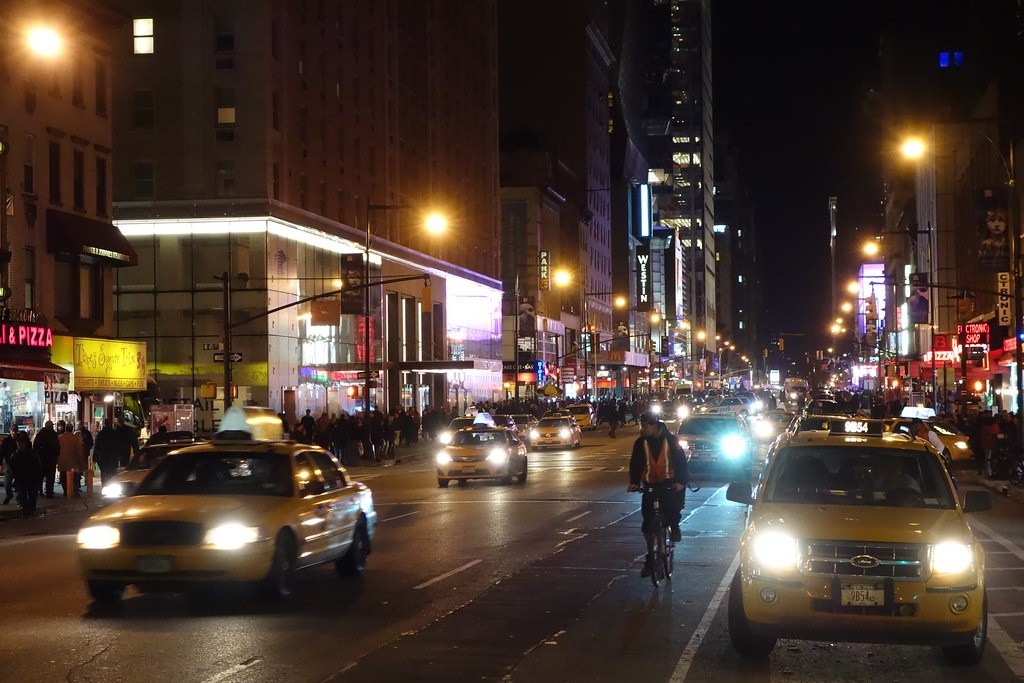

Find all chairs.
xmin=250 ymin=456 xmax=285 ymax=496
xmin=580 ymin=408 xmax=585 ymax=414
xmin=475 ymin=435 xmax=480 ymax=444
xmin=462 ymin=433 xmax=472 ymax=444
xmin=192 ymin=463 xmax=222 ymax=495
xmin=494 ymin=434 xmax=505 ymax=445
xmin=883 ymin=470 xmax=920 ymax=494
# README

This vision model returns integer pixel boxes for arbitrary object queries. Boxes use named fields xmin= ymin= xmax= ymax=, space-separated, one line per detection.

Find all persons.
xmin=471 ymin=392 xmax=694 ymax=439
xmin=1 ymin=416 xmax=169 ymax=519
xmin=627 ymin=411 xmax=689 ymax=578
xmin=279 ymin=404 xmax=460 ymax=466
xmin=796 ymin=379 xmax=1024 ymax=486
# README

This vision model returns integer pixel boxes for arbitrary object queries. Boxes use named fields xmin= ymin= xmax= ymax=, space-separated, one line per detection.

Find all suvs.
xmin=725 ymin=422 xmax=989 ymax=665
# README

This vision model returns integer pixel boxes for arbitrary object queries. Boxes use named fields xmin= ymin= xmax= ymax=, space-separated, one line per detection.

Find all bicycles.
xmin=627 ymin=484 xmax=677 ymax=587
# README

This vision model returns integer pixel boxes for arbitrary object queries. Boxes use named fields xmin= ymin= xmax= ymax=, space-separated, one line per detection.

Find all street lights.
xmin=830 ymin=267 xmax=901 ymax=382
xmin=582 ymin=288 xmax=748 ymax=405
xmin=513 ymin=262 xmax=572 ymax=417
xmin=364 ymin=201 xmax=448 ymax=463
xmin=864 ymin=227 xmax=938 ymax=412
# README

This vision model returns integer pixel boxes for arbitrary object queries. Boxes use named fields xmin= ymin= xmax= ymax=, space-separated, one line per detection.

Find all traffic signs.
xmin=213 ymin=352 xmax=242 ymax=363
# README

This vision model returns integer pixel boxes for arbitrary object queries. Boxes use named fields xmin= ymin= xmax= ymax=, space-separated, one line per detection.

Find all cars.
xmin=101 ymin=441 xmax=209 ymax=505
xmin=530 ymin=403 xmax=597 ymax=451
xmin=885 ymin=417 xmax=976 ymax=466
xmin=441 ymin=412 xmax=540 ymax=447
xmin=77 ymin=437 xmax=381 ymax=608
xmin=650 ymin=388 xmax=779 ymax=477
xmin=435 ymin=426 xmax=529 ymax=488
xmin=807 ymin=386 xmax=844 ymax=416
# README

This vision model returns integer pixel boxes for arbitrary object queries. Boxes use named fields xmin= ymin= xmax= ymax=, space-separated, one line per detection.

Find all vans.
xmin=783 ymin=378 xmax=808 ymax=404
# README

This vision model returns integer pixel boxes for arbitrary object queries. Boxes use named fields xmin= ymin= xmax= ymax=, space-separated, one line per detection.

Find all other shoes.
xmin=3 ymin=494 xmax=14 ymax=505
xmin=608 ymin=432 xmax=616 ymax=438
xmin=668 ymin=514 xmax=682 ymax=542
xmin=641 ymin=554 xmax=655 ymax=578
